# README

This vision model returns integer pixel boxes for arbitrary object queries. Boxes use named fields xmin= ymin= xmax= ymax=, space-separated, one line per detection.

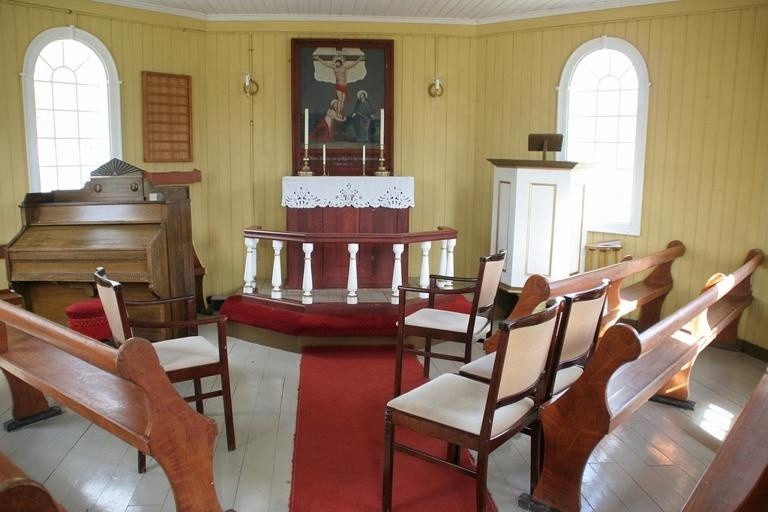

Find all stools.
xmin=587 ymin=237 xmax=624 ymax=270
xmin=65 ymin=298 xmax=112 ymax=342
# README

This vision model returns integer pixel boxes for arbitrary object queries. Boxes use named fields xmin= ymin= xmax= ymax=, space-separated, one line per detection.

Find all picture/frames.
xmin=142 ymin=71 xmax=193 ymax=163
xmin=288 ymin=36 xmax=394 ymax=176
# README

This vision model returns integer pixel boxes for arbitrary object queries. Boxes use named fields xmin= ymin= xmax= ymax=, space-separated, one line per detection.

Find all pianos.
xmin=1 ymin=171 xmax=199 ymax=343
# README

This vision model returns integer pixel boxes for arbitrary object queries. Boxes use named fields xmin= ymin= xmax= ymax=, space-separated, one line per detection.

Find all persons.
xmin=315 ymin=56 xmax=362 ymax=119
xmin=310 ymin=99 xmax=347 ymax=142
xmin=351 ymin=89 xmax=376 ymax=142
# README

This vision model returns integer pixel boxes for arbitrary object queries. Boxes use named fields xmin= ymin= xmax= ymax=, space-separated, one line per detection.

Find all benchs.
xmin=680 ymin=367 xmax=768 ymax=512
xmin=517 ymin=249 xmax=766 ymax=512
xmin=0 ymin=455 xmax=67 ymax=512
xmin=484 ymin=240 xmax=687 ymax=354
xmin=1 ymin=289 xmax=233 ymax=511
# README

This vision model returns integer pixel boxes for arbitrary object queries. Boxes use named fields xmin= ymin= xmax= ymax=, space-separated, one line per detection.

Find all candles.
xmin=363 ymin=144 xmax=365 ymax=164
xmin=305 ymin=108 xmax=310 ymax=149
xmin=322 ymin=144 xmax=326 ymax=164
xmin=379 ymin=108 xmax=385 ymax=147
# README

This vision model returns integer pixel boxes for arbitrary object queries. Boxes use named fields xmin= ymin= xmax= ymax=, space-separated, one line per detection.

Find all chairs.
xmin=458 ymin=277 xmax=611 ymax=474
xmin=382 ymin=301 xmax=565 ymax=511
xmin=93 ymin=266 xmax=237 ymax=472
xmin=393 ymin=249 xmax=507 ymax=398
